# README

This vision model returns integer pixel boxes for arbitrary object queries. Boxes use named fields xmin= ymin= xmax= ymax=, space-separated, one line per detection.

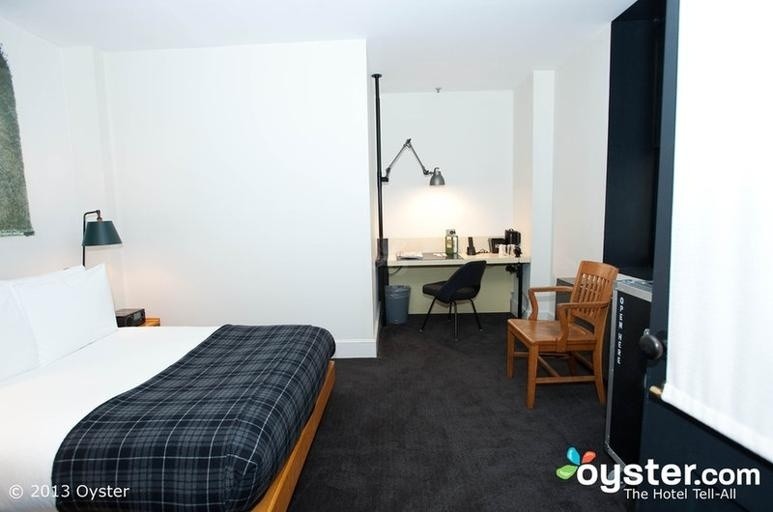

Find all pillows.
xmin=1 ymin=263 xmax=120 ymax=381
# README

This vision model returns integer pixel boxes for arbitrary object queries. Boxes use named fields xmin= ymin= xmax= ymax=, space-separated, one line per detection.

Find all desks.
xmin=387 ymin=251 xmax=530 ymax=319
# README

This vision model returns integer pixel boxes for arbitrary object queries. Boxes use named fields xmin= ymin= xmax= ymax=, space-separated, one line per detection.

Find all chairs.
xmin=418 ymin=260 xmax=487 ymax=337
xmin=507 ymin=260 xmax=620 ymax=410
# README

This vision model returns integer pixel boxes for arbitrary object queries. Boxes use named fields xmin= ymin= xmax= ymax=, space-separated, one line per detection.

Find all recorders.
xmin=114 ymin=308 xmax=145 ymax=327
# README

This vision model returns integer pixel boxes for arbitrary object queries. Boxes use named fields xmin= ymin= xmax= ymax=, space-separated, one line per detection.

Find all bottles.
xmin=444 ymin=228 xmax=459 ymax=256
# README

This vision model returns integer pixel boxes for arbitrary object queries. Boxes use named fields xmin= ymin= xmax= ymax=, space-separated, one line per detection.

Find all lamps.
xmin=81 ymin=210 xmax=122 ymax=266
xmin=381 ymin=138 xmax=445 ymax=186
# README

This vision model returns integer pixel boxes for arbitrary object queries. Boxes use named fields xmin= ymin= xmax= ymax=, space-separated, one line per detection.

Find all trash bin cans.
xmin=385 ymin=284 xmax=410 ymax=325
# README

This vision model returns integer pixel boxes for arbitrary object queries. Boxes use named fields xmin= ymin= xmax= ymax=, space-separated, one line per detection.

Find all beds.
xmin=0 ymin=263 xmax=336 ymax=510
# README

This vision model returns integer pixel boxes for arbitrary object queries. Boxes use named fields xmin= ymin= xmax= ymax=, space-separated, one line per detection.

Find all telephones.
xmin=467 ymin=237 xmax=476 ymax=255
